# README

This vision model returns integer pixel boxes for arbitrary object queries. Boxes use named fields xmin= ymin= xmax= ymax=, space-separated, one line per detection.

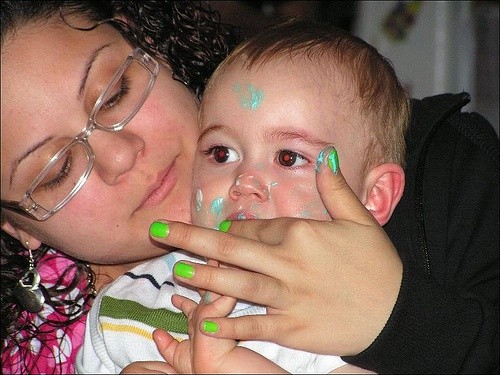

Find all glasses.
xmin=0 ymin=47 xmax=159 ymax=222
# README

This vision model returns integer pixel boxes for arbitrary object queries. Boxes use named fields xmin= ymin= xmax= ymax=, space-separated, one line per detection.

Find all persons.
xmin=0 ymin=0 xmax=500 ymax=375
xmin=75 ymin=17 xmax=410 ymax=375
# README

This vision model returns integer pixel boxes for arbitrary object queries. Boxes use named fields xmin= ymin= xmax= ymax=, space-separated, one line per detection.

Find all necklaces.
xmin=85 ymin=260 xmax=99 ymax=299
